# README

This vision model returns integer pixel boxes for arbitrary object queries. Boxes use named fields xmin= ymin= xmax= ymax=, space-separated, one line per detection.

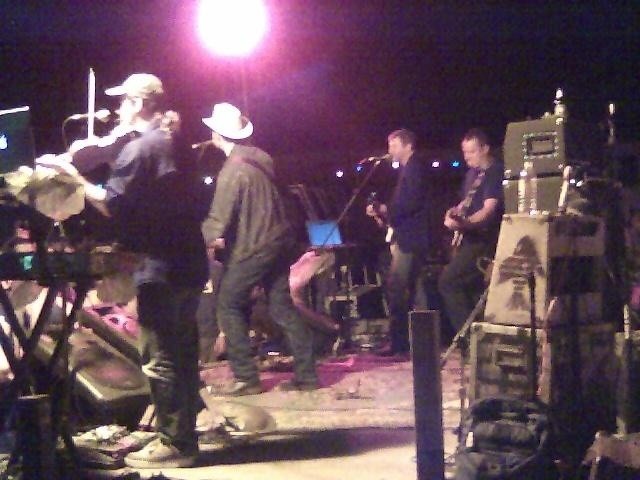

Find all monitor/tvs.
xmin=306 ymin=220 xmax=344 ymax=245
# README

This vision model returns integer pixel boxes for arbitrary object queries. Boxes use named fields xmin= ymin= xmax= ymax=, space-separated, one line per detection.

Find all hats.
xmin=103 ymin=72 xmax=166 ymax=104
xmin=200 ymin=102 xmax=254 ymax=140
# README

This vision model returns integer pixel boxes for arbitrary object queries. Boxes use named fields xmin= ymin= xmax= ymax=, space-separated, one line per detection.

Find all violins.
xmin=18 ymin=124 xmax=141 ymax=176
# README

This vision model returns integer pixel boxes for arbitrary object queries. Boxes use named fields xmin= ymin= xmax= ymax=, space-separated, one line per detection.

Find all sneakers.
xmin=220 ymin=378 xmax=264 ymax=397
xmin=280 ymin=375 xmax=321 ymax=392
xmin=375 ymin=340 xmax=411 ymax=362
xmin=122 ymin=433 xmax=201 ymax=469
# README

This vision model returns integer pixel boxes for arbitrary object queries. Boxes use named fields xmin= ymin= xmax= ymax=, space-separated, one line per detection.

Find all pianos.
xmin=0 ymin=195 xmax=113 ymax=243
xmin=0 ymin=250 xmax=145 ymax=282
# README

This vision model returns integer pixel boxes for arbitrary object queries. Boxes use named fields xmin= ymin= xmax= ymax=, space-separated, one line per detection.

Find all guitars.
xmin=451 ymin=197 xmax=471 ymax=247
xmin=367 ymin=191 xmax=395 ymax=245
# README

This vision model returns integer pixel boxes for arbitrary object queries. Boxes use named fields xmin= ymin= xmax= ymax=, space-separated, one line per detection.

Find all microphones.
xmin=368 ymin=153 xmax=393 ymax=161
xmin=70 ymin=108 xmax=111 ymax=124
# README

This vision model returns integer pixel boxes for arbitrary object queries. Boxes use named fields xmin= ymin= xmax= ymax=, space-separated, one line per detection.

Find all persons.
xmin=439 ymin=126 xmax=505 ymax=353
xmin=200 ymin=103 xmax=322 ymax=397
xmin=364 ymin=127 xmax=446 ymax=357
xmin=34 ymin=68 xmax=215 ymax=471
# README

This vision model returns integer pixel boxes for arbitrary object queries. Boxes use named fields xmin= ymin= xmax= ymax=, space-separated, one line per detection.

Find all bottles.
xmin=516 ymin=162 xmax=538 ymax=212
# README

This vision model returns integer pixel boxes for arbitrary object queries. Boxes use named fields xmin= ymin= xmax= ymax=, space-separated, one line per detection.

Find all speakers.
xmin=80 ymin=303 xmax=152 ymax=364
xmin=22 ymin=330 xmax=152 ymax=432
